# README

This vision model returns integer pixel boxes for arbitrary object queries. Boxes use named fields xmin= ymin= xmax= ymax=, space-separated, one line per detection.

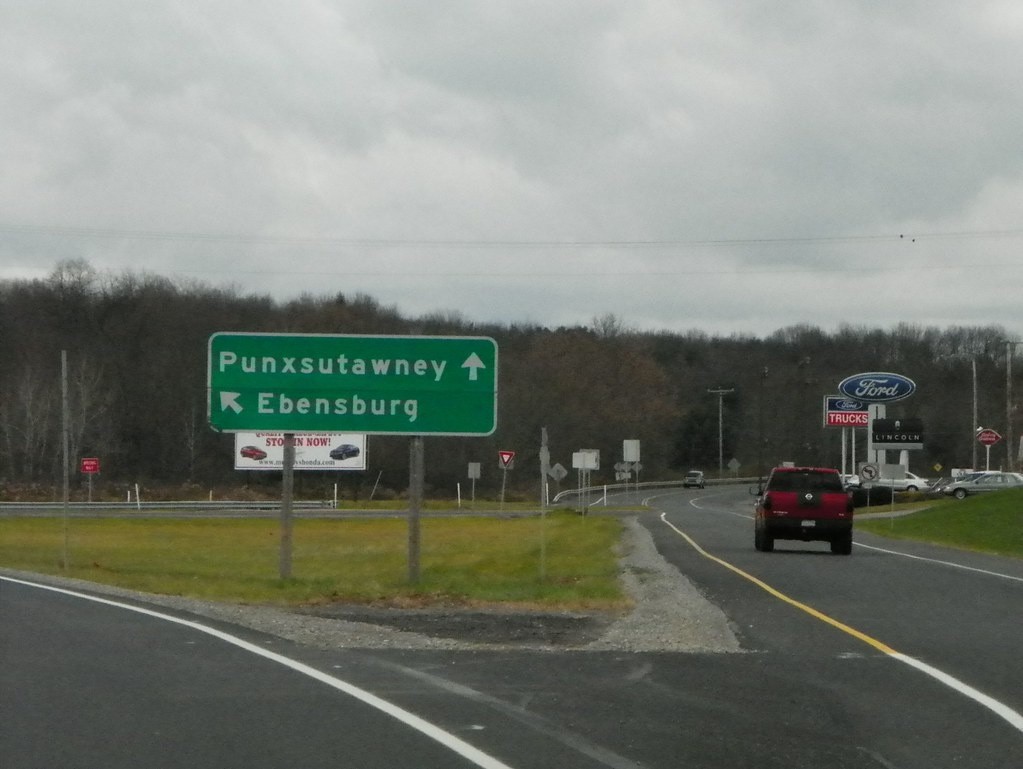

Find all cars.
xmin=839 ymin=474 xmax=860 ymax=490
xmin=846 ymin=469 xmax=933 ymax=493
xmin=240 ymin=445 xmax=267 ymax=461
xmin=329 ymin=444 xmax=360 ymax=460
xmin=942 ymin=471 xmax=1023 ymax=500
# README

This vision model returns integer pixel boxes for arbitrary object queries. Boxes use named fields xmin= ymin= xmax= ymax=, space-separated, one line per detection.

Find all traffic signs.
xmin=206 ymin=332 xmax=498 ymax=439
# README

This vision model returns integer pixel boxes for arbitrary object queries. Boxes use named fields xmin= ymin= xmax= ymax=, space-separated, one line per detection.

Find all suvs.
xmin=684 ymin=471 xmax=706 ymax=489
xmin=749 ymin=467 xmax=855 ymax=556
